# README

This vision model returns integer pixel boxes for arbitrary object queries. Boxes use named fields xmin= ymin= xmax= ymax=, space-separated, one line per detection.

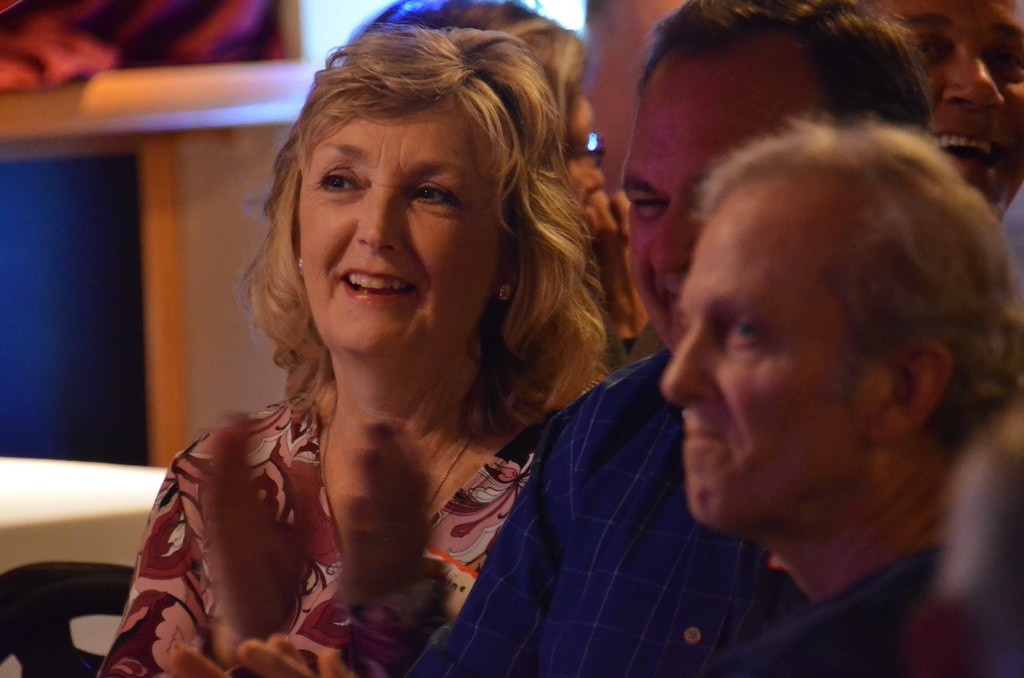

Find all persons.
xmin=96 ymin=0 xmax=1024 ymax=678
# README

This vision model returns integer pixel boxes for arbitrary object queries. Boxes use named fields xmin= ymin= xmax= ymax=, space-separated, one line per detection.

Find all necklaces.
xmin=323 ymin=392 xmax=471 ymax=543
